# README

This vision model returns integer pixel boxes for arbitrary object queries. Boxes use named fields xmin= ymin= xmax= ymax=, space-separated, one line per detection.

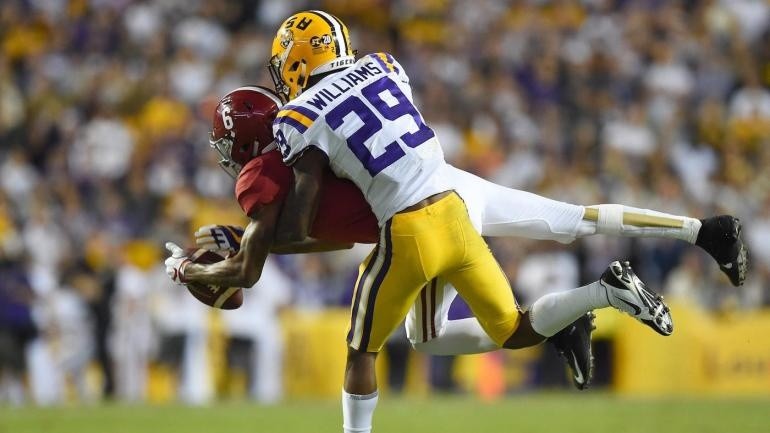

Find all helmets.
xmin=208 ymin=85 xmax=286 ymax=167
xmin=271 ymin=8 xmax=357 ymax=104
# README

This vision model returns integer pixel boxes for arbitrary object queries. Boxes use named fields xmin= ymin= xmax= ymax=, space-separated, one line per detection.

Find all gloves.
xmin=192 ymin=224 xmax=245 ymax=260
xmin=163 ymin=242 xmax=194 ymax=286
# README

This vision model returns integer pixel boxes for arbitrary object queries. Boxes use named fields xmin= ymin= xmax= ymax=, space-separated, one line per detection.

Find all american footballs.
xmin=180 ymin=246 xmax=244 ymax=311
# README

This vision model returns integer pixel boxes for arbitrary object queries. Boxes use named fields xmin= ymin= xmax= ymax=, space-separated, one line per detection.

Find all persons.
xmin=2 ymin=0 xmax=769 ymax=398
xmin=266 ymin=8 xmax=676 ymax=433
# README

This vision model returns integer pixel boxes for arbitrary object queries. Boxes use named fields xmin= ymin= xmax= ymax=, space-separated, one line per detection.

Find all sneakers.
xmin=601 ymin=260 xmax=674 ymax=337
xmin=695 ymin=214 xmax=748 ymax=287
xmin=549 ymin=307 xmax=596 ymax=390
